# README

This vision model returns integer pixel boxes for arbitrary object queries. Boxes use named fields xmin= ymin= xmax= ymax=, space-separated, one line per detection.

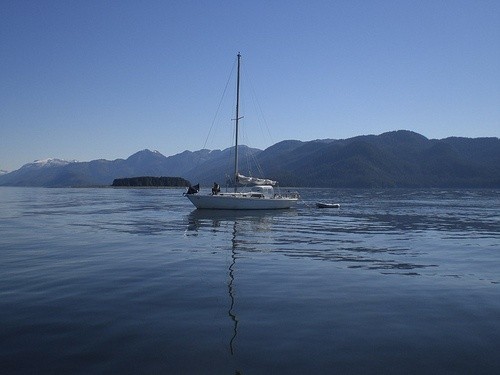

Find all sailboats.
xmin=182 ymin=51 xmax=299 ymax=210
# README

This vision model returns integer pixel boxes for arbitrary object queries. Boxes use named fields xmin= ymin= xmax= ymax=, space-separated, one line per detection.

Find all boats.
xmin=316 ymin=202 xmax=340 ymax=208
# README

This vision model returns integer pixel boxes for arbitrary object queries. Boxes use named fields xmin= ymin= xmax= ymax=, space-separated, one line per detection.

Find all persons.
xmin=212 ymin=182 xmax=221 ymax=195
